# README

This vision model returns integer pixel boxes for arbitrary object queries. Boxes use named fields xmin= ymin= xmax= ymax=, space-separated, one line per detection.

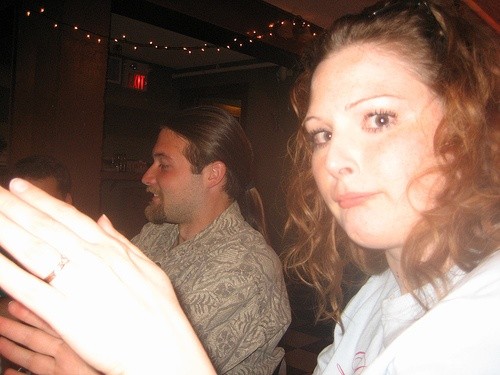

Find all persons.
xmin=0 ymin=106 xmax=293 ymax=375
xmin=0 ymin=0 xmax=500 ymax=375
xmin=5 ymin=153 xmax=73 ymax=206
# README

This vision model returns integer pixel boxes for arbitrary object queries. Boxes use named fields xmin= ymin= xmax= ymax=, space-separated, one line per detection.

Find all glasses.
xmin=363 ymin=0 xmax=445 ymax=34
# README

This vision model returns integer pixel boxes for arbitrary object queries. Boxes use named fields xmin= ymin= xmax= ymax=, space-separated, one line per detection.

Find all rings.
xmin=44 ymin=257 xmax=68 ymax=283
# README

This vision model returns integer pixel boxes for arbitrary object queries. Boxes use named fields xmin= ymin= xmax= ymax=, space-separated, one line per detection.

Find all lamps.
xmin=128 ymin=62 xmax=151 ymax=91
